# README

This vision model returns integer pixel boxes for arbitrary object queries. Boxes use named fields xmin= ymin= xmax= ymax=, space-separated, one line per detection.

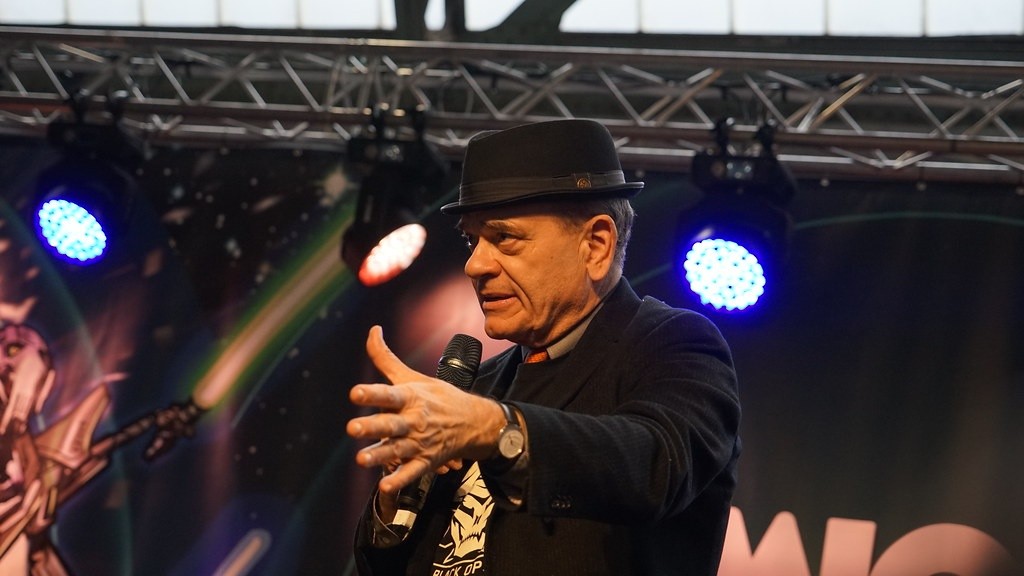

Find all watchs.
xmin=493 ymin=400 xmax=526 ymax=469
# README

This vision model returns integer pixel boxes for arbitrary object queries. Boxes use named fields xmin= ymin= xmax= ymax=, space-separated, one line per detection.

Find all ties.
xmin=524 ymin=347 xmax=547 ymax=364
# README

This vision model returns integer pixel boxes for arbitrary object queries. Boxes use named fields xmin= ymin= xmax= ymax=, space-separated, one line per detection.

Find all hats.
xmin=439 ymin=115 xmax=646 ymax=217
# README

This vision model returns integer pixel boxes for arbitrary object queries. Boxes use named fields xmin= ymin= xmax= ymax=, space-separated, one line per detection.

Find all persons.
xmin=345 ymin=117 xmax=741 ymax=576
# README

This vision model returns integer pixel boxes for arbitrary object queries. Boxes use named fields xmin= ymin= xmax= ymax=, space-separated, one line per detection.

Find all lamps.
xmin=32 ymin=123 xmax=148 ymax=274
xmin=341 ymin=200 xmax=430 ymax=288
xmin=677 ymin=198 xmax=780 ymax=324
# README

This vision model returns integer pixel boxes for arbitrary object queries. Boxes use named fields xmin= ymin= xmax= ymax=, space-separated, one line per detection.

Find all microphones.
xmin=390 ymin=333 xmax=484 ymax=541
